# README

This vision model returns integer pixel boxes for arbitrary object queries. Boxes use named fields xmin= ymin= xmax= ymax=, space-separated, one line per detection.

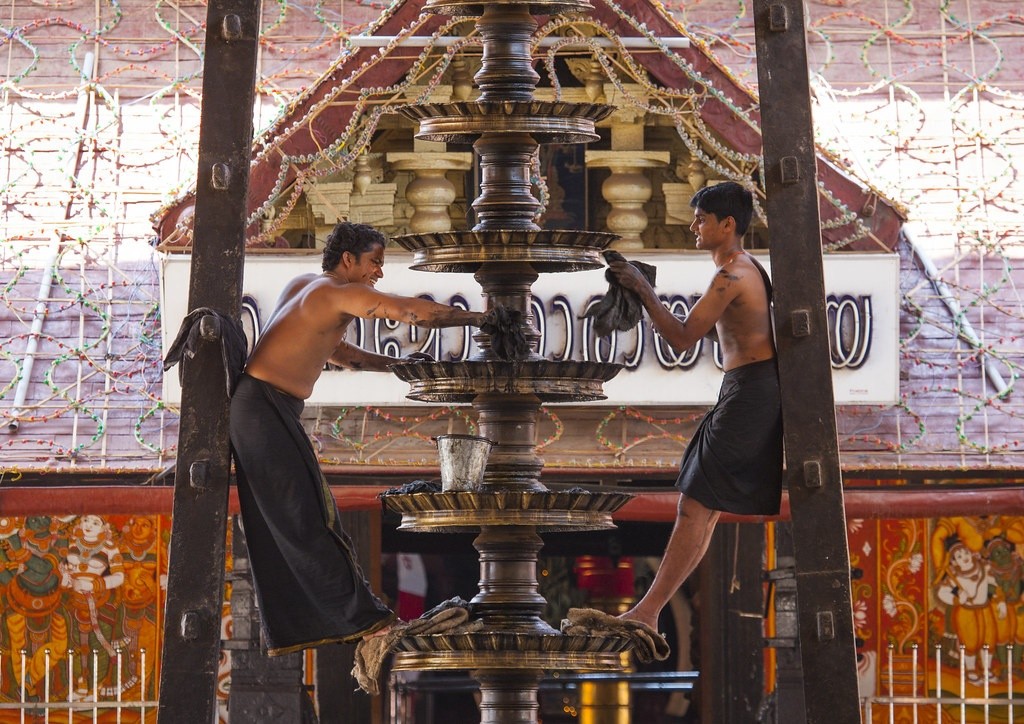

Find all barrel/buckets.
xmin=431 ymin=434 xmax=498 ymax=493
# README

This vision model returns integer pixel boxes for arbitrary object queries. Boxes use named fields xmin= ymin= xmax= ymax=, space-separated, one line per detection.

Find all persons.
xmin=231 ymin=221 xmax=499 ymax=659
xmin=610 ymin=182 xmax=783 ymax=635
